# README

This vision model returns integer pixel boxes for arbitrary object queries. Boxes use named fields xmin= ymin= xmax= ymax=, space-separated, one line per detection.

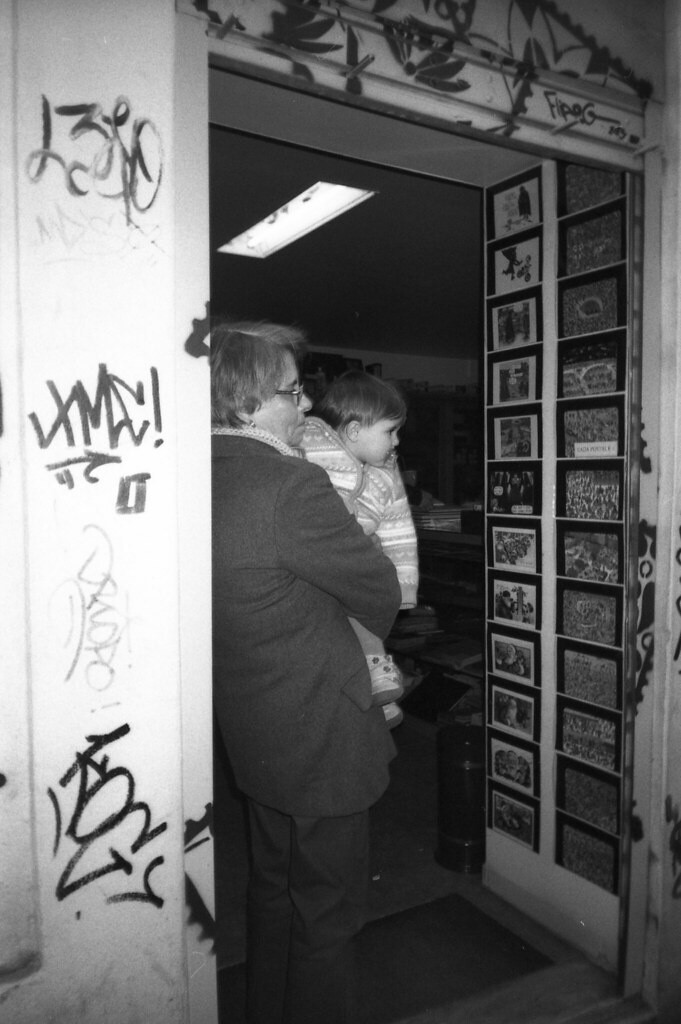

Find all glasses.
xmin=272 ymin=384 xmax=304 ymax=405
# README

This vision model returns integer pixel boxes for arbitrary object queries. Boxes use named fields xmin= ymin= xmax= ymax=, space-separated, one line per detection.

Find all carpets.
xmin=216 ymin=893 xmax=555 ymax=1024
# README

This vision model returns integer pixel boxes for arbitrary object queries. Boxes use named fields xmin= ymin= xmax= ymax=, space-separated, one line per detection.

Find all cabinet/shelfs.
xmin=404 ymin=526 xmax=487 ymax=744
xmin=400 ymin=390 xmax=484 ymax=506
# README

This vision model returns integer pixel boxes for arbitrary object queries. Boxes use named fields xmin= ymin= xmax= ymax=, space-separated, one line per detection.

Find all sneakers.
xmin=366 ymin=654 xmax=404 ymax=705
xmin=382 ymin=701 xmax=404 ymax=730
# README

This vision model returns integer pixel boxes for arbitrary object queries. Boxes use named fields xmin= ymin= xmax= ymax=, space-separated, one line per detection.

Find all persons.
xmin=211 ymin=317 xmax=403 ymax=1024
xmin=498 ymin=304 xmax=529 ymax=344
xmin=517 ymin=186 xmax=530 ymax=223
xmin=404 ymin=481 xmax=435 ymax=510
xmin=497 ymin=534 xmax=535 ymax=562
xmin=300 ymin=368 xmax=419 ymax=726
xmin=501 ymin=645 xmax=525 ymax=674
xmin=496 ymin=588 xmax=533 ymax=623
xmin=494 ymin=749 xmax=530 ymax=785
xmin=499 ymin=801 xmax=531 ymax=829
xmin=511 ymin=420 xmax=524 ymax=448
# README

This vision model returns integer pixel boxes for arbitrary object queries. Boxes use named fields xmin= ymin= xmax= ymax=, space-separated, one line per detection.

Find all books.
xmin=388 ymin=505 xmax=485 ymax=728
